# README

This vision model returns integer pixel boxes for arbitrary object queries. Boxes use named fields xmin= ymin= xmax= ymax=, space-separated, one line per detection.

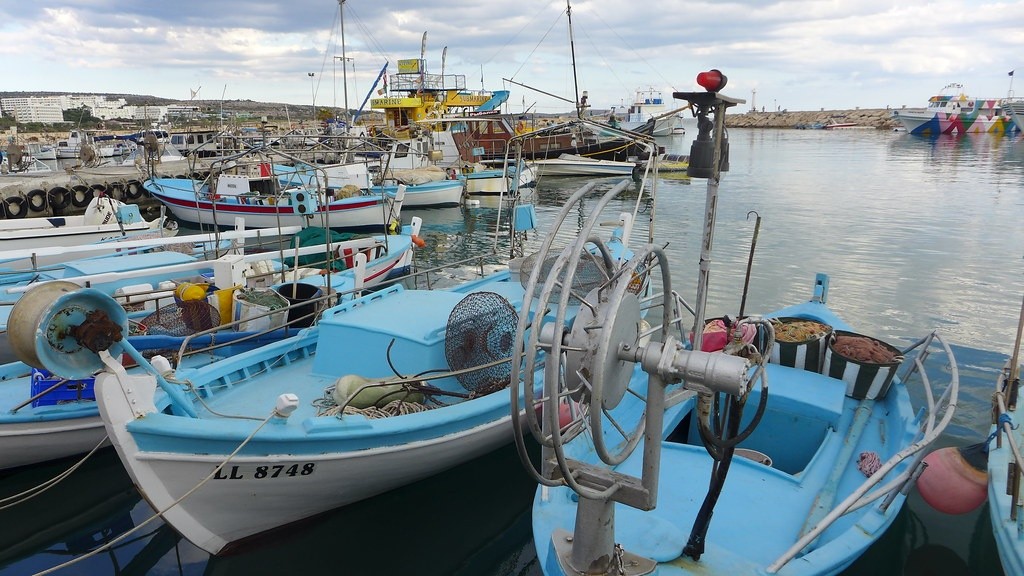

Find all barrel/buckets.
xmin=278 ymin=282 xmax=323 ymax=328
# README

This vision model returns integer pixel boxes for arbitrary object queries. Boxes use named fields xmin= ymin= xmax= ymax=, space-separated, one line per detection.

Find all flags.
xmin=1008 ymin=71 xmax=1014 ymax=76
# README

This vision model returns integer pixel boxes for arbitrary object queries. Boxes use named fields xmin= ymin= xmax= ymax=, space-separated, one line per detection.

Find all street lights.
xmin=307 ymin=72 xmax=316 ymax=124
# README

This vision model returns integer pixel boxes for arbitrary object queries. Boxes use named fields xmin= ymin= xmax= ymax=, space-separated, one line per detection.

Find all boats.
xmin=986 ymin=340 xmax=1024 ymax=576
xmin=0 ymin=4 xmax=695 ymax=554
xmin=893 ymin=80 xmax=1016 ymax=135
xmin=503 ymin=65 xmax=960 ymax=576
